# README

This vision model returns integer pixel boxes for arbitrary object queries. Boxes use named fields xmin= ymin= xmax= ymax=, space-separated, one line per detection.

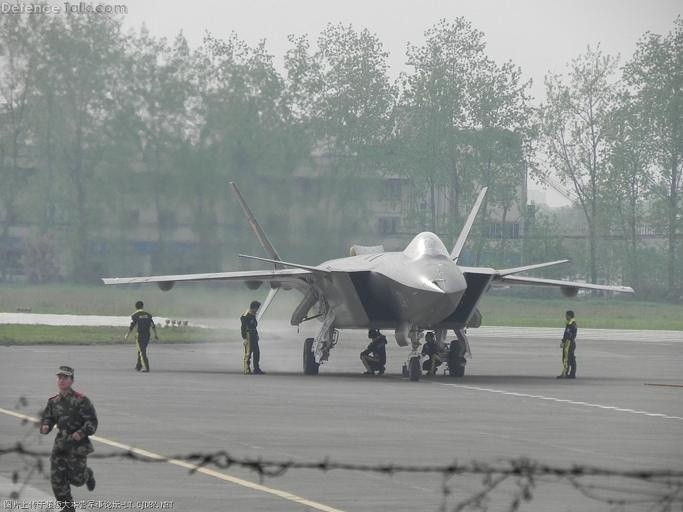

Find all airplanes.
xmin=99 ymin=179 xmax=638 ymax=383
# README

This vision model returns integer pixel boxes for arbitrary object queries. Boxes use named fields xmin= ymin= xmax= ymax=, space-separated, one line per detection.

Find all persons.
xmin=40 ymin=366 xmax=98 ymax=512
xmin=360 ymin=329 xmax=387 ymax=374
xmin=421 ymin=332 xmax=444 ymax=378
xmin=557 ymin=311 xmax=577 ymax=378
xmin=240 ymin=301 xmax=264 ymax=375
xmin=124 ymin=301 xmax=159 ymax=372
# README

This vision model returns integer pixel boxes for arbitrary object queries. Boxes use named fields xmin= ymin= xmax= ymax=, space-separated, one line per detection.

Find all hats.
xmin=56 ymin=366 xmax=74 ymax=376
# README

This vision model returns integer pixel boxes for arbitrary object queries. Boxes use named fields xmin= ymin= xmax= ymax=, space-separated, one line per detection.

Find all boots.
xmin=557 ymin=376 xmax=576 ymax=379
xmin=58 ymin=507 xmax=75 ymax=512
xmin=135 ymin=368 xmax=149 ymax=372
xmin=85 ymin=468 xmax=95 ymax=492
xmin=254 ymin=369 xmax=265 ymax=374
xmin=364 ymin=367 xmax=385 ymax=376
